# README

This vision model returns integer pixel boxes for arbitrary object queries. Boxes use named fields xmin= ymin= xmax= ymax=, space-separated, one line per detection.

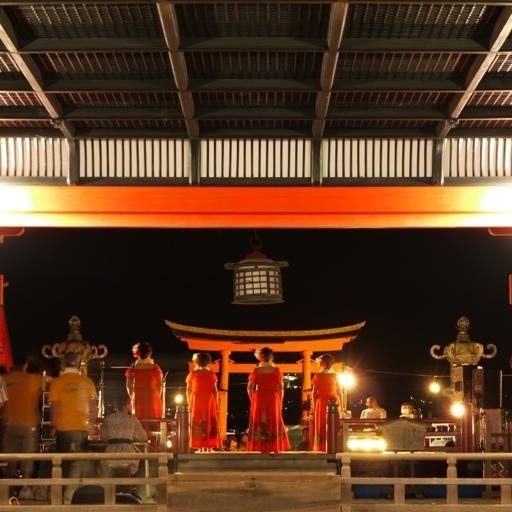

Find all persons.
xmin=399 ymin=403 xmax=416 ymax=418
xmin=123 ymin=340 xmax=164 ymax=453
xmin=89 ymin=420 xmax=103 ymax=441
xmin=47 ymin=350 xmax=98 ymax=504
xmin=247 ymin=346 xmax=293 ymax=456
xmin=340 ymin=397 xmax=353 ymax=419
xmin=0 ymin=353 xmax=50 ymax=497
xmin=99 ymin=392 xmax=149 ymax=497
xmin=223 ymin=427 xmax=309 ymax=452
xmin=186 ymin=352 xmax=223 ymax=453
xmin=306 ymin=352 xmax=345 ymax=452
xmin=358 ymin=395 xmax=388 ymax=420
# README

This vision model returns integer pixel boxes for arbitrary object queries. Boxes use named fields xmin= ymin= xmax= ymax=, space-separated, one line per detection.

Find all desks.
xmin=47 ymin=440 xmax=151 ymax=496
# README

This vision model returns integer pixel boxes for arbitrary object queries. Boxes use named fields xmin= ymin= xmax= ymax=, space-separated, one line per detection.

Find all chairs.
xmin=101 ymin=439 xmax=146 ymax=504
